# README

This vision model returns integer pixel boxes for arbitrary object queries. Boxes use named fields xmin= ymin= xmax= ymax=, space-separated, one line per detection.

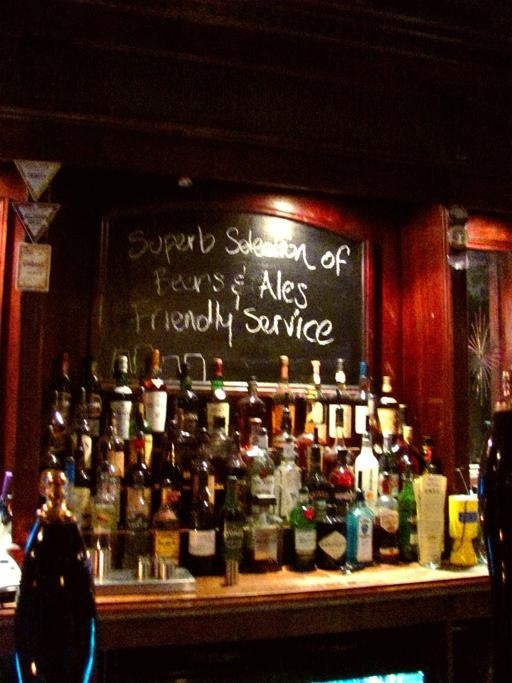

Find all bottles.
xmin=36 ymin=347 xmax=448 ymax=571
xmin=0 ymin=468 xmax=12 ymax=552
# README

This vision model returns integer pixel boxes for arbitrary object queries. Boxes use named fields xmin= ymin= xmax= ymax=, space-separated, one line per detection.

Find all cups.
xmin=137 ymin=555 xmax=178 ymax=579
xmin=448 ymin=494 xmax=481 ymax=567
xmin=225 ymin=560 xmax=239 ymax=587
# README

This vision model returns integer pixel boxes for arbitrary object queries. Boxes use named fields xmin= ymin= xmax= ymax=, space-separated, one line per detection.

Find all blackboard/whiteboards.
xmin=88 ymin=192 xmax=376 ymax=405
